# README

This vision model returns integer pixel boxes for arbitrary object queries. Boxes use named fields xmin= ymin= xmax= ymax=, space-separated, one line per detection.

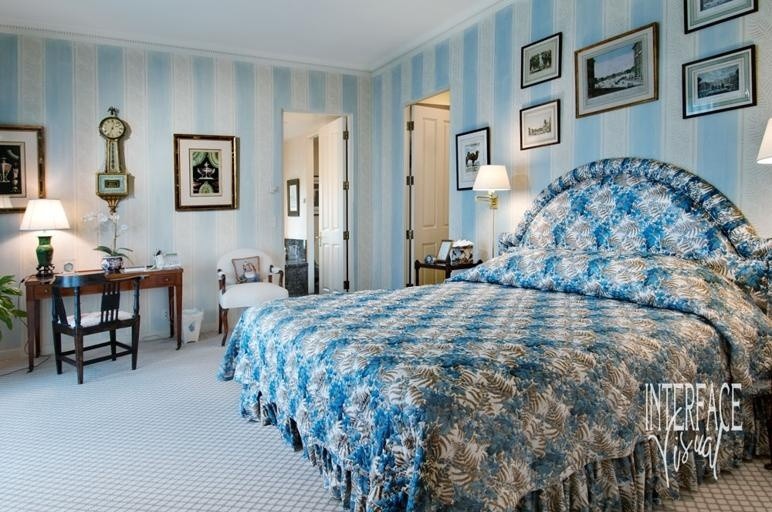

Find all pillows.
xmin=231 ymin=255 xmax=263 ymax=283
xmin=468 ymin=243 xmax=710 ymax=311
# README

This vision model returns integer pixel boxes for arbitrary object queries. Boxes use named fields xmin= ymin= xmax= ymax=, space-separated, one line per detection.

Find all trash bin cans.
xmin=181 ymin=309 xmax=203 ymax=345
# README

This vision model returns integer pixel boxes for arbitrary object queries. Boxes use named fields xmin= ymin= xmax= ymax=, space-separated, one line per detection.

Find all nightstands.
xmin=412 ymin=255 xmax=483 ymax=284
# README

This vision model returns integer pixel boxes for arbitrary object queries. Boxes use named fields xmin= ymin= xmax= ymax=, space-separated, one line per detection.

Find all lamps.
xmin=757 ymin=117 xmax=772 ymax=175
xmin=473 ymin=162 xmax=512 ymax=257
xmin=19 ymin=196 xmax=70 ymax=277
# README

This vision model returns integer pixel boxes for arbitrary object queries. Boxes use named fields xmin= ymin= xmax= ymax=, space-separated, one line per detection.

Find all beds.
xmin=218 ymin=155 xmax=770 ymax=512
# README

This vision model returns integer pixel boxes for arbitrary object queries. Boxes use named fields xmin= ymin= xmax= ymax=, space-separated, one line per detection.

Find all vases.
xmin=99 ymin=256 xmax=124 ymax=273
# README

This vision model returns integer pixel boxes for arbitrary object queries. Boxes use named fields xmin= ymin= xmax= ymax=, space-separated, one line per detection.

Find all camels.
xmin=465 ymin=150 xmax=479 ymax=167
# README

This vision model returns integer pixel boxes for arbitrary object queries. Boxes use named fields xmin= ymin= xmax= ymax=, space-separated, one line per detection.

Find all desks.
xmin=20 ymin=265 xmax=188 ymax=374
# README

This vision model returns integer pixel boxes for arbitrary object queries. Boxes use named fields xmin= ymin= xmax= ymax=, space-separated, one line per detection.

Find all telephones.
xmin=157 ymin=252 xmax=180 ymax=270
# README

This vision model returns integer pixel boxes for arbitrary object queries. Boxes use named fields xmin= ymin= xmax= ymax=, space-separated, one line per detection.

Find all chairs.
xmin=215 ymin=248 xmax=289 ymax=348
xmin=48 ymin=271 xmax=147 ymax=384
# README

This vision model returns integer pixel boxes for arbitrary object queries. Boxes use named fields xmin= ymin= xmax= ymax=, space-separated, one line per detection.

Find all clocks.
xmin=93 ymin=103 xmax=129 ymax=214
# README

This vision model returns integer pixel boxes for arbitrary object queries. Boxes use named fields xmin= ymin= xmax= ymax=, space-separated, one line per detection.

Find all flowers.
xmin=82 ymin=207 xmax=137 ymax=262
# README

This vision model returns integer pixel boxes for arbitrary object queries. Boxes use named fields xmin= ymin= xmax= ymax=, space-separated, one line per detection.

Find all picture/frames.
xmin=286 ymin=177 xmax=303 ymax=217
xmin=172 ymin=132 xmax=239 ymax=213
xmin=682 ymin=41 xmax=757 ymax=119
xmin=570 ymin=20 xmax=661 ymax=119
xmin=518 ymin=30 xmax=562 ymax=92
xmin=0 ymin=122 xmax=47 ymax=215
xmin=682 ymin=0 xmax=758 ymax=35
xmin=518 ymin=98 xmax=562 ymax=150
xmin=455 ymin=125 xmax=492 ymax=193
